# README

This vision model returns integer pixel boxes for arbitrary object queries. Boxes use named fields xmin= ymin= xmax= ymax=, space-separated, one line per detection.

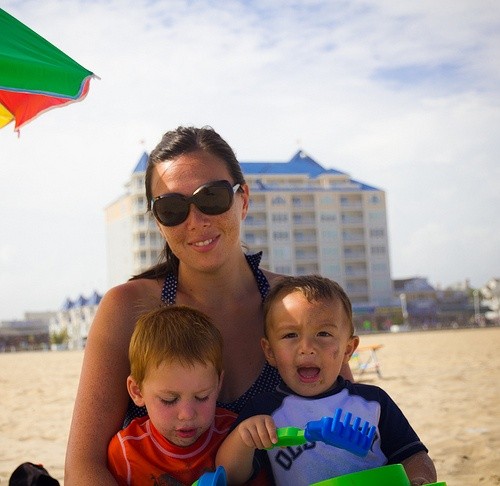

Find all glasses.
xmin=148 ymin=180 xmax=241 ymax=226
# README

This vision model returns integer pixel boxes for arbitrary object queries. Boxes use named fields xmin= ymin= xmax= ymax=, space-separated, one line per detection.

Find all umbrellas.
xmin=0 ymin=9 xmax=99 ymax=136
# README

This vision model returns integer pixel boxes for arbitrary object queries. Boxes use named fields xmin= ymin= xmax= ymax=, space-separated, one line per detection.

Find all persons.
xmin=105 ymin=305 xmax=254 ymax=486
xmin=217 ymin=273 xmax=437 ymax=486
xmin=64 ymin=127 xmax=353 ymax=486
